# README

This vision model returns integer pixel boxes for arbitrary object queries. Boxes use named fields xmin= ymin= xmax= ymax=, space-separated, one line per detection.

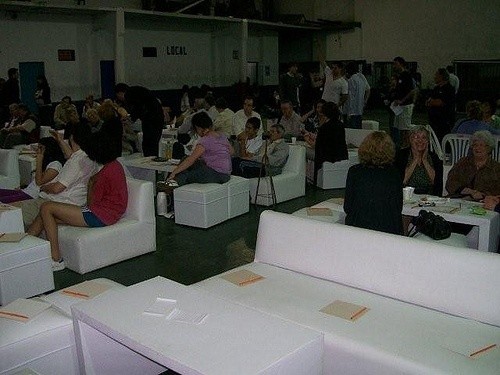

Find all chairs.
xmin=490 ymin=138 xmax=500 ymax=162
xmin=428 ymin=126 xmax=443 ymax=160
xmin=442 ymin=132 xmax=473 ymax=165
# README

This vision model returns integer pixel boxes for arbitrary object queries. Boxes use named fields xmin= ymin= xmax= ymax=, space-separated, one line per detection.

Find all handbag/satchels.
xmin=407 ymin=209 xmax=451 ymax=240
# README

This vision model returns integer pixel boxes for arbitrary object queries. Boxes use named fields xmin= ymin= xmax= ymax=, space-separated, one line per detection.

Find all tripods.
xmin=254 ymin=139 xmax=277 ymax=210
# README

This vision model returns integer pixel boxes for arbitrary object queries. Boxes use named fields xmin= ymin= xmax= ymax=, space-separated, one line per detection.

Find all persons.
xmin=0 ymin=54 xmax=500 ymax=271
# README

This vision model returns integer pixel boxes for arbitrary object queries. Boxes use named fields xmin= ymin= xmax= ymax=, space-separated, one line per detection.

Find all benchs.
xmin=0 ymin=121 xmax=500 ymax=375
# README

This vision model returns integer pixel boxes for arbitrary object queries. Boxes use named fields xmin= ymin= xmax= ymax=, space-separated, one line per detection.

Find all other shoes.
xmin=52 ymin=257 xmax=66 ymax=272
xmin=398 ymin=144 xmax=409 ymax=149
xmin=159 ymin=179 xmax=178 ymax=187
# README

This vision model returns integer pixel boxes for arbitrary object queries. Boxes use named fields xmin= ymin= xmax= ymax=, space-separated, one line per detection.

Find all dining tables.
xmin=132 ymin=156 xmax=181 ymax=171
xmin=70 ymin=276 xmax=324 ymax=375
xmin=402 ymin=193 xmax=500 ymax=253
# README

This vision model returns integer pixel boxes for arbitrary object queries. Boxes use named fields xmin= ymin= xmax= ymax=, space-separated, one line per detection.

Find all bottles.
xmin=156 ymin=190 xmax=167 ymax=216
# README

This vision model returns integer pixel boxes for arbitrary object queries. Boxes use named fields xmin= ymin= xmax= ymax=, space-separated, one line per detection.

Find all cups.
xmin=403 ymin=187 xmax=415 ymax=200
xmin=291 ymin=137 xmax=296 ymax=143
xmin=40 ymin=126 xmax=54 ymax=139
xmin=167 ymin=125 xmax=170 ymax=129
